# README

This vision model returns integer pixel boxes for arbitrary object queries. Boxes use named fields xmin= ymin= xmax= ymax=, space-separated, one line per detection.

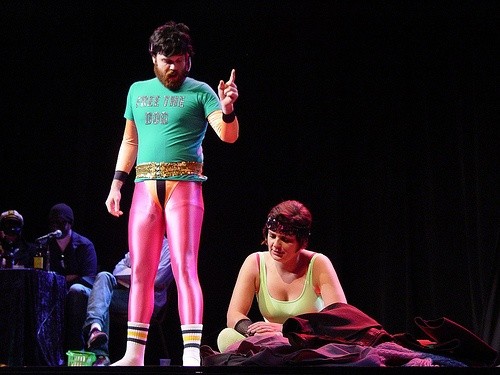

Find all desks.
xmin=0 ymin=267 xmax=66 ymax=368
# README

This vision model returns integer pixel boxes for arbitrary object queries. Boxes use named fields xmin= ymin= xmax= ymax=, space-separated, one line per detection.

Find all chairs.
xmin=113 ymin=280 xmax=174 ymax=362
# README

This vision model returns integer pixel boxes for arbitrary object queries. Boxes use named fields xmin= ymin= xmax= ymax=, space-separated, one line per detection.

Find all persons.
xmin=65 ymin=235 xmax=173 ymax=366
xmin=0 ymin=209 xmax=24 ymax=267
xmin=30 ymin=203 xmax=97 ymax=351
xmin=217 ymin=201 xmax=349 ymax=354
xmin=106 ymin=24 xmax=240 ymax=366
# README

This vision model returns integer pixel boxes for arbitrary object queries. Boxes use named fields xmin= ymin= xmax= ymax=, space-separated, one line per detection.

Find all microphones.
xmin=33 ymin=230 xmax=62 ymax=243
